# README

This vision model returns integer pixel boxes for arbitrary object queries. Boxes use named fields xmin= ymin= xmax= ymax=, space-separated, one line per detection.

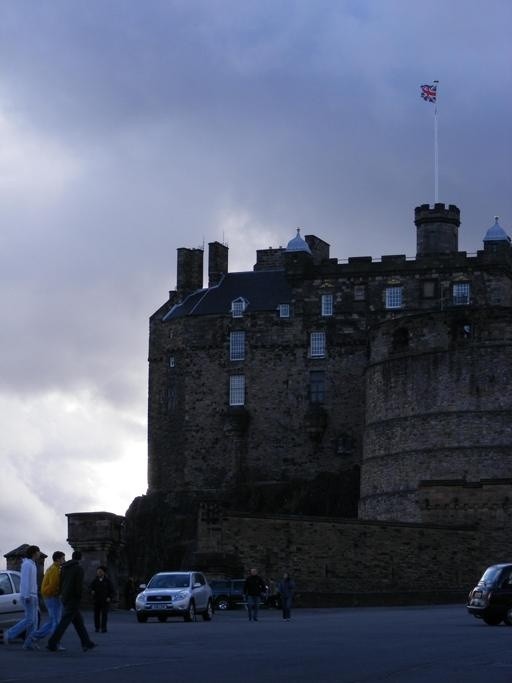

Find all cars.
xmin=465 ymin=563 xmax=512 ymax=626
xmin=1 ymin=569 xmax=41 ymax=641
xmin=133 ymin=569 xmax=214 ymax=623
xmin=209 ymin=579 xmax=265 ymax=610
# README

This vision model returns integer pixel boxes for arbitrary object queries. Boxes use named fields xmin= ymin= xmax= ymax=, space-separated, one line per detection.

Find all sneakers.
xmin=248 ymin=617 xmax=257 ymax=622
xmin=48 ymin=640 xmax=67 ymax=651
xmin=23 ymin=640 xmax=40 ymax=650
xmin=283 ymin=618 xmax=290 ymax=622
xmin=3 ymin=631 xmax=8 ymax=646
xmin=81 ymin=640 xmax=98 ymax=652
xmin=95 ymin=628 xmax=107 ymax=633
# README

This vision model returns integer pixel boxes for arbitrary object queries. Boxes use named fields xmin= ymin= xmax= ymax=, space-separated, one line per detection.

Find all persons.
xmin=242 ymin=567 xmax=265 ymax=623
xmin=2 ymin=544 xmax=41 ymax=650
xmin=21 ymin=551 xmax=68 ymax=652
xmin=278 ymin=572 xmax=298 ymax=625
xmin=89 ymin=565 xmax=117 ymax=634
xmin=46 ymin=550 xmax=99 ymax=652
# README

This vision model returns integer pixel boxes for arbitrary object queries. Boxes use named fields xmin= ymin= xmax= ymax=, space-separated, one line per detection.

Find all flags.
xmin=419 ymin=83 xmax=438 ymax=103
